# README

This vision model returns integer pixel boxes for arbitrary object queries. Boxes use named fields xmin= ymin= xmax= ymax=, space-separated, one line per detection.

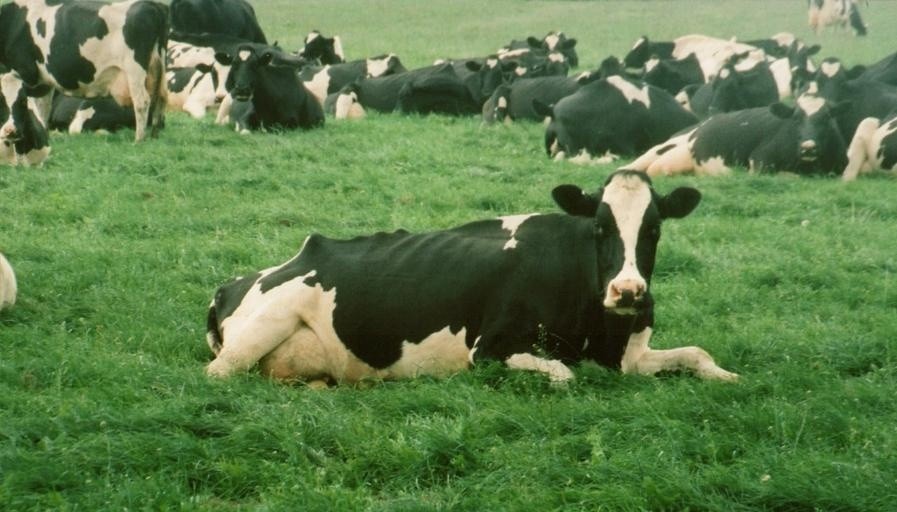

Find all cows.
xmin=200 ymin=169 xmax=743 ymax=391
xmin=0 ymin=0 xmax=897 ymax=184
